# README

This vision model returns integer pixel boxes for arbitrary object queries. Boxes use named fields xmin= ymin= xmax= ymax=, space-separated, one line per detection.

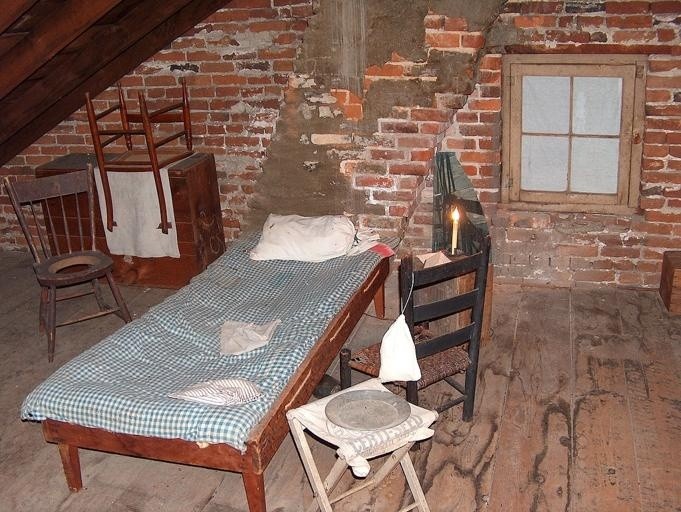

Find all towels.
xmin=93 ymin=167 xmax=182 ymax=260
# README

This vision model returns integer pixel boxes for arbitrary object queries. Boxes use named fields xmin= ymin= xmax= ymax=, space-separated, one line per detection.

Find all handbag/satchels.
xmin=377 ymin=272 xmax=422 ymax=384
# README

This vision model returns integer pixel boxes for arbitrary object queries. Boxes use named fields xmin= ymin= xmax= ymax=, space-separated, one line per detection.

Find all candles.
xmin=451 ymin=221 xmax=460 ymax=256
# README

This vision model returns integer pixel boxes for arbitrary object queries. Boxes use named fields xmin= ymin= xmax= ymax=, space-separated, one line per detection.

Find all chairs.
xmin=338 ymin=235 xmax=492 ymax=452
xmin=85 ymin=77 xmax=194 ymax=235
xmin=6 ymin=162 xmax=133 ymax=364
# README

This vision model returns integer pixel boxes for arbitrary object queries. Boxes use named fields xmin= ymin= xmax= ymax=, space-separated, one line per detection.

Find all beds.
xmin=41 ymin=228 xmax=390 ymax=511
xmin=249 ymin=212 xmax=355 ymax=264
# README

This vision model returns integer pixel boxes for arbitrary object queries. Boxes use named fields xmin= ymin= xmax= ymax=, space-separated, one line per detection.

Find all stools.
xmin=285 ymin=377 xmax=440 ymax=512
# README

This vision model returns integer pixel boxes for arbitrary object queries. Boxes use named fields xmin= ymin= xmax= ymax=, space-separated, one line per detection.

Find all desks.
xmin=35 ymin=153 xmax=227 ymax=290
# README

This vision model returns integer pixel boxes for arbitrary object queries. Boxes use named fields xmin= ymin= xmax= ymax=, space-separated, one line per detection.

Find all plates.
xmin=325 ymin=391 xmax=412 ymax=432
